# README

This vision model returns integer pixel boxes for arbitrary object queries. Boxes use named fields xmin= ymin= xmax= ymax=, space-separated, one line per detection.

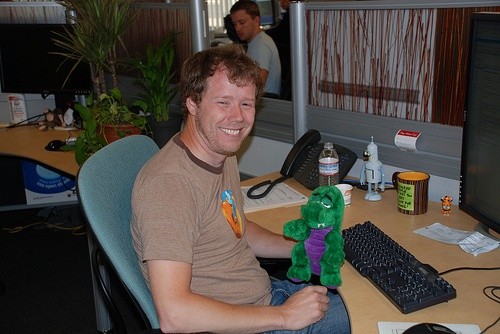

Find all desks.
xmin=0 ymin=121 xmax=96 ymax=181
xmin=239 ymin=167 xmax=500 ymax=334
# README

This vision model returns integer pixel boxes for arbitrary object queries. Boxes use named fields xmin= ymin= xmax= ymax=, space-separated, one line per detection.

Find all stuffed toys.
xmin=283 ymin=185 xmax=346 ymax=289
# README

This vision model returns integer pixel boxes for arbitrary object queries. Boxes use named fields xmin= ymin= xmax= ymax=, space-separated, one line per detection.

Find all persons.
xmin=130 ymin=44 xmax=351 ymax=334
xmin=230 ymin=0 xmax=281 ymax=97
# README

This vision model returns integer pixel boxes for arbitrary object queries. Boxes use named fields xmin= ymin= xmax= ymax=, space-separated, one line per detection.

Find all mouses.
xmin=402 ymin=322 xmax=458 ymax=334
xmin=47 ymin=140 xmax=66 ymax=148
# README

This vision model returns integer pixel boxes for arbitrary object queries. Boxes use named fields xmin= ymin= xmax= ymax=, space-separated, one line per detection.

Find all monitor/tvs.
xmin=459 ymin=12 xmax=500 ymax=244
xmin=0 ymin=23 xmax=98 ymax=130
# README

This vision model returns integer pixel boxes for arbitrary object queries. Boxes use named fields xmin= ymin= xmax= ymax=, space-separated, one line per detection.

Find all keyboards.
xmin=342 ymin=220 xmax=457 ymax=314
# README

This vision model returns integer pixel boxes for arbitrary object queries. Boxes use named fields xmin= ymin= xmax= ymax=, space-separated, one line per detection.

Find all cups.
xmin=335 ymin=184 xmax=353 ymax=208
xmin=392 ymin=171 xmax=430 ymax=215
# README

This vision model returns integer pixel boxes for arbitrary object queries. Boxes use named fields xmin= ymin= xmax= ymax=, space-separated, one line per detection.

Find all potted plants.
xmin=120 ymin=28 xmax=184 ymax=150
xmin=48 ymin=0 xmax=145 ymax=167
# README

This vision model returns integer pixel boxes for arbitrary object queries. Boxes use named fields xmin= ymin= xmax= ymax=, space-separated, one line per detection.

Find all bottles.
xmin=318 ymin=142 xmax=339 ymax=187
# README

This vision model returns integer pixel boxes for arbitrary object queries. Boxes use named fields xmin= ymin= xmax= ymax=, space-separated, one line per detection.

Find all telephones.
xmin=280 ymin=130 xmax=358 ymax=191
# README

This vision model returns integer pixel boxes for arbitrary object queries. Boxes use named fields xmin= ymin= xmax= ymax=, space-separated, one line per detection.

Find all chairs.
xmin=74 ymin=134 xmax=162 ymax=334
